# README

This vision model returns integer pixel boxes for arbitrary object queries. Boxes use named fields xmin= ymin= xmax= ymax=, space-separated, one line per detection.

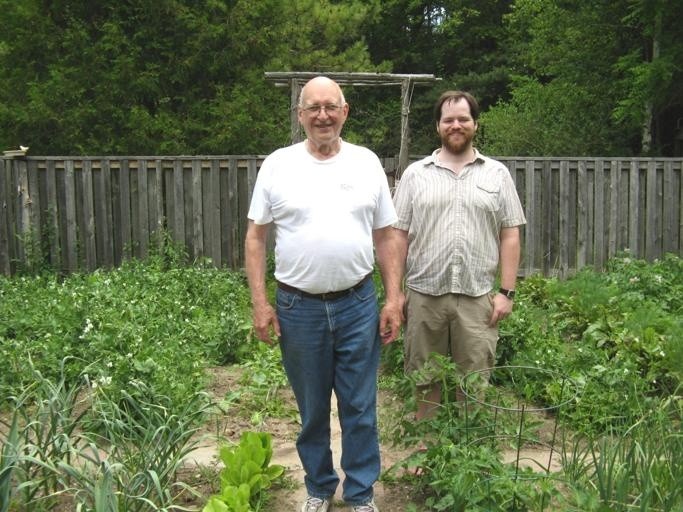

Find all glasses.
xmin=303 ymin=104 xmax=344 ymax=113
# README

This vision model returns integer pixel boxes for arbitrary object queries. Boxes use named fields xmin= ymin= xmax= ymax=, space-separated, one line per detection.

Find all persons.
xmin=392 ymin=91 xmax=527 ymax=474
xmin=245 ymin=76 xmax=401 ymax=512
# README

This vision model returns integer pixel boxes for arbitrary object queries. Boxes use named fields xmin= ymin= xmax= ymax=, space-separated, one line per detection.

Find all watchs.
xmin=499 ymin=288 xmax=515 ymax=297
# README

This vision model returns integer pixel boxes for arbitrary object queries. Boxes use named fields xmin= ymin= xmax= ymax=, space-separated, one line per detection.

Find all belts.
xmin=278 ymin=273 xmax=372 ymax=301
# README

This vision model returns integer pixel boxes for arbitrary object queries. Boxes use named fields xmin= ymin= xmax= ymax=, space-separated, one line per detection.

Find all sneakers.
xmin=302 ymin=495 xmax=380 ymax=512
